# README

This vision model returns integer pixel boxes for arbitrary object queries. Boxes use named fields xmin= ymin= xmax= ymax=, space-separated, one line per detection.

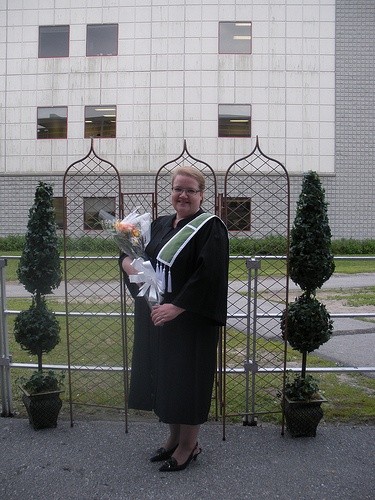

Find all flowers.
xmin=97 ymin=214 xmax=170 ymax=328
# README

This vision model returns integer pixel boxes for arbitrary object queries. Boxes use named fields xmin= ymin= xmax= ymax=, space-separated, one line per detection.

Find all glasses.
xmin=172 ymin=186 xmax=202 ymax=196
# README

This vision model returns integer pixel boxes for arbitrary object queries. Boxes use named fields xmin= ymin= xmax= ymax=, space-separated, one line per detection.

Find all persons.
xmin=119 ymin=166 xmax=230 ymax=471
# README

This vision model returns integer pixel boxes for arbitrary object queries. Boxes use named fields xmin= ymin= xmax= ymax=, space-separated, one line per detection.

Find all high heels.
xmin=150 ymin=441 xmax=202 ymax=471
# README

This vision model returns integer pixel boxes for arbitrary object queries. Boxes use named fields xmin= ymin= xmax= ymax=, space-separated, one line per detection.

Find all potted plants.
xmin=280 ymin=169 xmax=336 ymax=440
xmin=16 ymin=182 xmax=63 ymax=431
